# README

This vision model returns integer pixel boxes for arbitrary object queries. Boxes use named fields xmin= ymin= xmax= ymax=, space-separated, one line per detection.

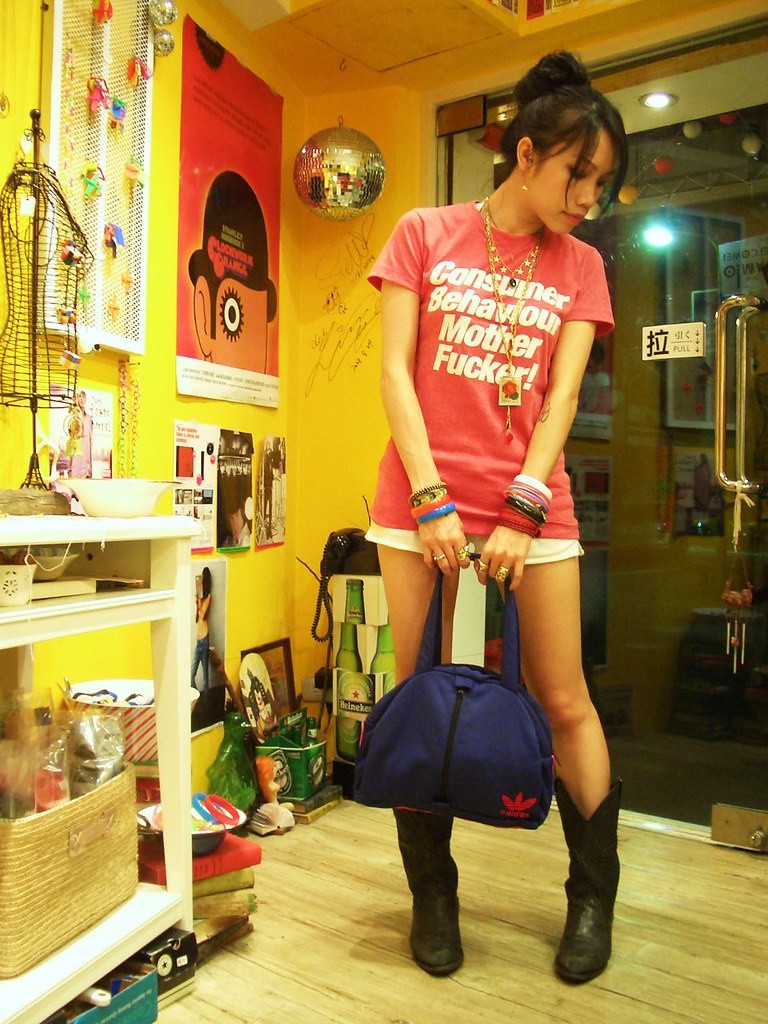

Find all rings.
xmin=453 ymin=546 xmax=468 ymax=562
xmin=496 ymin=563 xmax=509 ymax=583
xmin=473 ymin=558 xmax=489 ymax=575
xmin=431 ymin=553 xmax=446 ymax=562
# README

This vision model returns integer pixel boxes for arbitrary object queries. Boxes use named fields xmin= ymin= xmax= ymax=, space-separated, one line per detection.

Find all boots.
xmin=552 ymin=778 xmax=621 ymax=983
xmin=391 ymin=807 xmax=464 ymax=973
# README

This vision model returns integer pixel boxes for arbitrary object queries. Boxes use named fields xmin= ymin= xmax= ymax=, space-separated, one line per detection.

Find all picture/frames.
xmin=240 ymin=636 xmax=296 ymax=719
xmin=662 ymin=205 xmax=746 ymax=432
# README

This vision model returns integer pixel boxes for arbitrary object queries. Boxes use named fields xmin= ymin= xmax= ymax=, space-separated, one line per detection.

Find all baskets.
xmin=1 ymin=755 xmax=138 ymax=982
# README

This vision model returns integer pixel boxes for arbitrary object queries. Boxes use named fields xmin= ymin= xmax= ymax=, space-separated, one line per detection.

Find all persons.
xmin=365 ymin=48 xmax=626 ymax=987
xmin=191 ymin=566 xmax=213 ymax=721
xmin=105 ymin=224 xmax=116 ymax=258
xmin=222 ymin=498 xmax=251 ymax=550
xmin=265 ymin=438 xmax=282 ymax=540
xmin=61 ymin=391 xmax=92 ymax=479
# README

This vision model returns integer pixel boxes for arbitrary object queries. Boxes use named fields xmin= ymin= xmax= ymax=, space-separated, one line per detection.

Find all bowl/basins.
xmin=136 ymin=802 xmax=247 ymax=857
xmin=0 ymin=564 xmax=37 ymax=607
xmin=56 ymin=478 xmax=183 ymax=517
xmin=63 ymin=679 xmax=201 ymax=762
xmin=33 ymin=552 xmax=80 ymax=580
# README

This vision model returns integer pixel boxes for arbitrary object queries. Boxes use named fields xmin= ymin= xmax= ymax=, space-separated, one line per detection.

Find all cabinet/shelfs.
xmin=0 ymin=516 xmax=202 ymax=1024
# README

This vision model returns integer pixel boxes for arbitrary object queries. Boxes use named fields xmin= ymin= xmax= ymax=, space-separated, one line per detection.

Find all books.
xmin=281 ymin=783 xmax=345 ymax=826
xmin=248 ymin=803 xmax=296 ymax=836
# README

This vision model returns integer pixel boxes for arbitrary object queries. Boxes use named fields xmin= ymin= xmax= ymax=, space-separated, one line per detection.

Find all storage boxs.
xmin=255 ymin=739 xmax=328 ymax=802
xmin=59 ymin=927 xmax=200 ymax=1024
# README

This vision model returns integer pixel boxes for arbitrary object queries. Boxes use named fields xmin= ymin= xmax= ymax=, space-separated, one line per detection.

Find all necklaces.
xmin=484 ymin=198 xmax=544 ymax=437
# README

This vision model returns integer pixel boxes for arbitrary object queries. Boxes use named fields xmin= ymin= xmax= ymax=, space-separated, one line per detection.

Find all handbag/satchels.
xmin=353 ymin=552 xmax=556 ymax=831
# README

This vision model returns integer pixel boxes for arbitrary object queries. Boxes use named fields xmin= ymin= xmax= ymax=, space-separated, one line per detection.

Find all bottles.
xmin=291 ymin=717 xmax=319 ymax=748
xmin=335 ymin=579 xmax=395 ymax=762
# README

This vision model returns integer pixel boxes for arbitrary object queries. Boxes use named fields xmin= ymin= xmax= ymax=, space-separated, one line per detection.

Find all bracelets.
xmin=497 ymin=474 xmax=552 ymax=540
xmin=191 ymin=792 xmax=240 ymax=824
xmin=408 ymin=481 xmax=455 ymax=526
